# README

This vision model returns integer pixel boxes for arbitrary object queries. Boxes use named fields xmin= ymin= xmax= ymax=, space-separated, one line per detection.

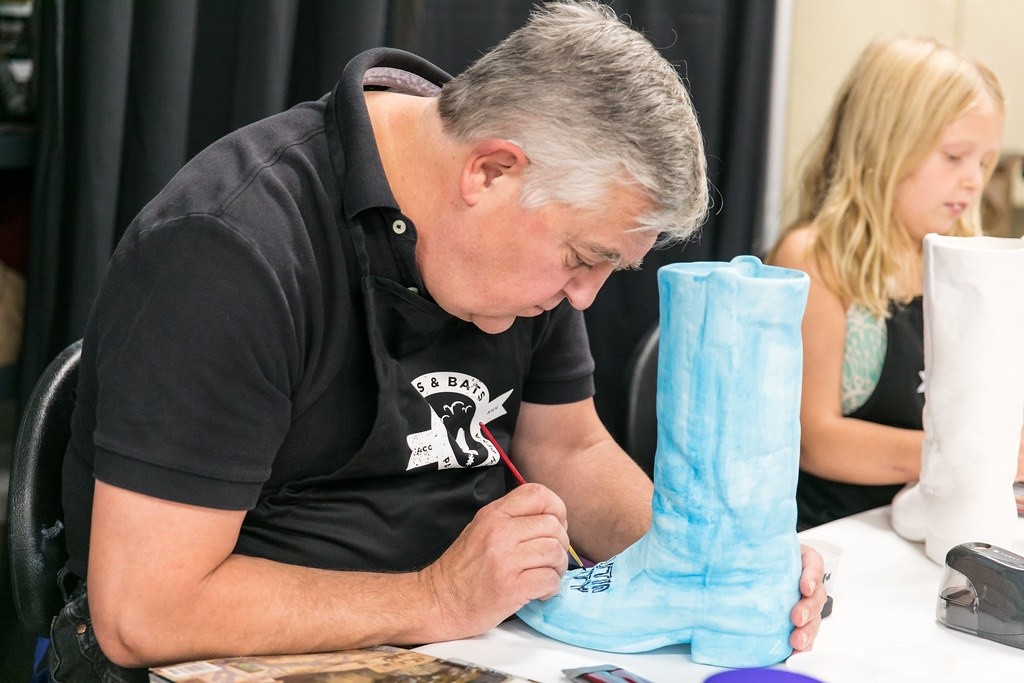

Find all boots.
xmin=891 ymin=234 xmax=1024 ymax=566
xmin=514 ymin=252 xmax=810 ymax=669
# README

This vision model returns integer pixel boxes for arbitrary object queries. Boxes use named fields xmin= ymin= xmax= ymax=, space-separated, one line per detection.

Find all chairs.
xmin=6 ymin=337 xmax=82 ymax=637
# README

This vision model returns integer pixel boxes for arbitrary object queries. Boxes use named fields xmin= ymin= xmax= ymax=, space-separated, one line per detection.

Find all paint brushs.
xmin=477 ymin=421 xmax=588 ymax=572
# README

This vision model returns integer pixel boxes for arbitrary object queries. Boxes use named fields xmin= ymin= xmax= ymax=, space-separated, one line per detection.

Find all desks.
xmin=152 ymin=507 xmax=1024 ymax=683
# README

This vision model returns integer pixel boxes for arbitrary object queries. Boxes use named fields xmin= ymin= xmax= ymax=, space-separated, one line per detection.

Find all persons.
xmin=46 ymin=0 xmax=828 ymax=682
xmin=767 ymin=34 xmax=1023 ymax=531
